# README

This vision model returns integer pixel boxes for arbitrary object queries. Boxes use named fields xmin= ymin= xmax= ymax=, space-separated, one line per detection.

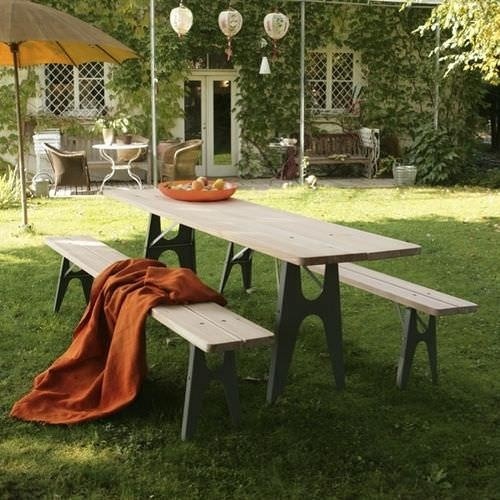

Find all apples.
xmin=198 ymin=177 xmax=208 ymax=186
xmin=192 ymin=180 xmax=203 ymax=190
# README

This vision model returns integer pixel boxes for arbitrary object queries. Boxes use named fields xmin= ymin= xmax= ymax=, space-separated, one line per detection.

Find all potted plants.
xmin=92 ymin=111 xmax=151 ymax=144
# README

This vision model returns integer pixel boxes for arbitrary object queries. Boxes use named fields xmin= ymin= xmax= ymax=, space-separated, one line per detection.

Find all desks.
xmin=104 ymin=191 xmax=421 ymax=404
xmin=92 ymin=143 xmax=149 ymax=195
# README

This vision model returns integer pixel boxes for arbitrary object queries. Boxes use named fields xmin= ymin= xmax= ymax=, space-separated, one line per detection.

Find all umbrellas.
xmin=0 ymin=0 xmax=145 ymax=226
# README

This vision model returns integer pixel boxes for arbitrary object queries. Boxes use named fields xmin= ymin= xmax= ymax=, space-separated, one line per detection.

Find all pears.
xmin=213 ymin=179 xmax=225 ymax=189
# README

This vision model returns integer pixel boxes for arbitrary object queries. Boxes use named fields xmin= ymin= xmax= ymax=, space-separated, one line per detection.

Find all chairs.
xmin=43 ymin=143 xmax=91 ymax=195
xmin=160 ymin=139 xmax=203 ymax=183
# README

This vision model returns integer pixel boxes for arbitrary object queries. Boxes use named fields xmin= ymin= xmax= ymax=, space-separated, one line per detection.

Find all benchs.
xmin=220 ymin=241 xmax=478 ymax=391
xmin=44 ymin=235 xmax=277 ymax=442
xmin=288 ymin=133 xmax=375 ymax=180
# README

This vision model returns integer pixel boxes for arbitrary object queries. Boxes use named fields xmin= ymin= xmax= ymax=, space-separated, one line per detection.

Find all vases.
xmin=392 ymin=166 xmax=416 ymax=187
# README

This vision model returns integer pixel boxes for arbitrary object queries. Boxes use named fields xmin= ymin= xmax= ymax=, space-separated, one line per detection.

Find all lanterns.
xmin=170 ymin=6 xmax=193 ymax=35
xmin=264 ymin=11 xmax=289 ymax=40
xmin=218 ymin=8 xmax=243 ymax=37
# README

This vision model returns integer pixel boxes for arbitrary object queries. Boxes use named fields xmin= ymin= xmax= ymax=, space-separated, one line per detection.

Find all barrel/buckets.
xmin=394 ymin=164 xmax=416 ymax=186
